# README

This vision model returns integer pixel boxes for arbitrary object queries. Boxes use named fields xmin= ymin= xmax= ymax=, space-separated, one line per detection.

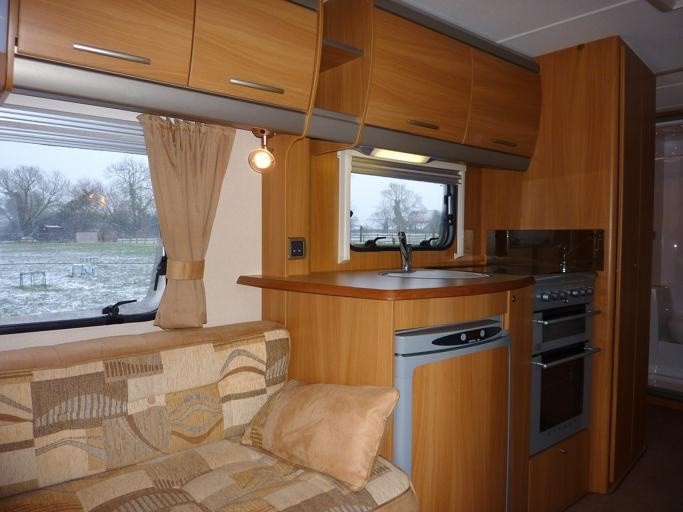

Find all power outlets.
xmin=288 ymin=237 xmax=305 ymax=259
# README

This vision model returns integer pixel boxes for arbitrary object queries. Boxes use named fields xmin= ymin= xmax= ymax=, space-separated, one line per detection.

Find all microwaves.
xmin=482 ymin=229 xmax=604 ymax=276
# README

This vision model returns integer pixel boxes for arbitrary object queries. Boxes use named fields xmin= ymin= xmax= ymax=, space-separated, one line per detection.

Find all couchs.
xmin=0 ymin=320 xmax=421 ymax=512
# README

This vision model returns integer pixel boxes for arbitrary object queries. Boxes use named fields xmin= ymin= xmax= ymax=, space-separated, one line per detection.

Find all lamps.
xmin=248 ymin=128 xmax=276 ymax=174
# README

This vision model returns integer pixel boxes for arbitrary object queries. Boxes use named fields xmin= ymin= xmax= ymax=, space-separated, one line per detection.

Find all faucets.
xmin=398 ymin=231 xmax=412 ymax=272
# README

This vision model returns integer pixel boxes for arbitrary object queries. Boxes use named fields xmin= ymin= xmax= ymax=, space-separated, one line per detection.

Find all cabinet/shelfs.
xmin=470 ymin=34 xmax=655 ymax=494
xmin=6 ymin=0 xmax=326 ymax=138
xmin=529 ymin=429 xmax=590 ymax=511
xmin=310 ymin=0 xmax=541 ymax=175
xmin=262 ymin=288 xmax=534 ymax=512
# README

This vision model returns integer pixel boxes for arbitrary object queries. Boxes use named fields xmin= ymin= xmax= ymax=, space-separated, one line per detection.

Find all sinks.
xmin=381 ymin=269 xmax=490 ymax=279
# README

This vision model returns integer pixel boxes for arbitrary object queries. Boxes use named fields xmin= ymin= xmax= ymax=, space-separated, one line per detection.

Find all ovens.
xmin=527 ymin=304 xmax=601 ymax=455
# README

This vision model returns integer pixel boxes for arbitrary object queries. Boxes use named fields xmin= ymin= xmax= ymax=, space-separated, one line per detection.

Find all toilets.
xmin=648 ymin=284 xmax=683 ymax=378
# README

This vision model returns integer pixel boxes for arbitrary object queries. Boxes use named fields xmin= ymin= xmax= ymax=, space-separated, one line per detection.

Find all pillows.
xmin=240 ymin=377 xmax=401 ymax=492
